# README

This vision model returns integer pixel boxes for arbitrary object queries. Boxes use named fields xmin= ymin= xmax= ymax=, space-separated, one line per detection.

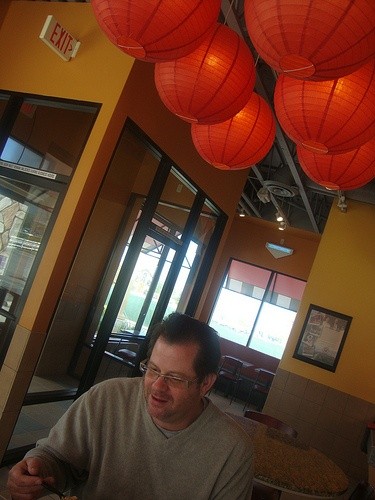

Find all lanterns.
xmin=154 ymin=24 xmax=256 ymax=125
xmin=244 ymin=0 xmax=375 ymax=82
xmin=191 ymin=91 xmax=276 ymax=170
xmin=296 ymin=138 xmax=375 ymax=191
xmin=274 ymin=59 xmax=375 ymax=154
xmin=90 ymin=0 xmax=221 ymax=63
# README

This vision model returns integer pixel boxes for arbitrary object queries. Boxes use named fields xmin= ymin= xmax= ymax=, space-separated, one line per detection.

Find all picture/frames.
xmin=293 ymin=303 xmax=354 ymax=373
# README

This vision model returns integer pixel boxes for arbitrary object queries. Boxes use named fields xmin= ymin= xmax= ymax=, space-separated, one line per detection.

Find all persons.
xmin=7 ymin=313 xmax=255 ymax=500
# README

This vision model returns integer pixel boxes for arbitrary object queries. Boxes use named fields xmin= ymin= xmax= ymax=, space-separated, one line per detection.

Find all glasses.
xmin=140 ymin=359 xmax=203 ymax=389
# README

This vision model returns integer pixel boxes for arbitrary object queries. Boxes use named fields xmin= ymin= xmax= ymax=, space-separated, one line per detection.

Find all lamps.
xmin=274 ymin=209 xmax=286 ymax=230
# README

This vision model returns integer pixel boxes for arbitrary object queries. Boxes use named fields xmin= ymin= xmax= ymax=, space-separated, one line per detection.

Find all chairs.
xmin=241 ymin=368 xmax=276 ymax=413
xmin=209 ymin=355 xmax=246 ymax=405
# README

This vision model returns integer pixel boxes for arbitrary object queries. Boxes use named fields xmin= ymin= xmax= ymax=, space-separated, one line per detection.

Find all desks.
xmin=224 ymin=412 xmax=350 ymax=500
xmin=225 ymin=357 xmax=253 ymax=396
